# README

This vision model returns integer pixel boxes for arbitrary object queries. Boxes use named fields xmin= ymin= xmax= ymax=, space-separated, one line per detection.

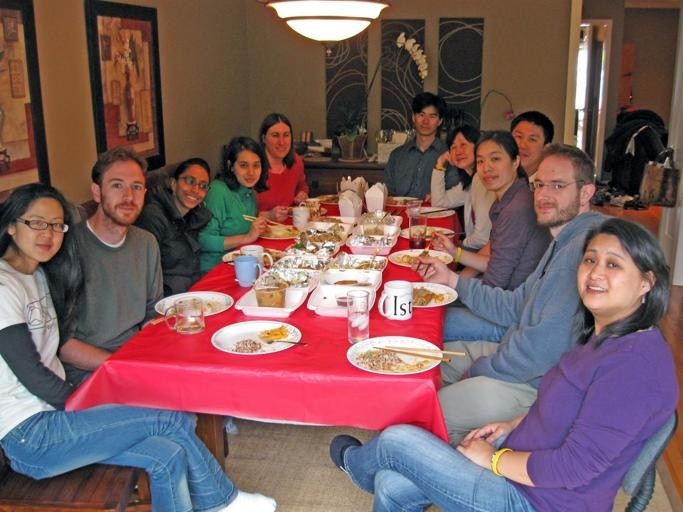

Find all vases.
xmin=337 ymin=131 xmax=366 ymax=160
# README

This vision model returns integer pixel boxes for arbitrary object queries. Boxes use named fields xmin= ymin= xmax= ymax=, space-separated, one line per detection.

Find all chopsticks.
xmin=372 ymin=342 xmax=467 ymax=366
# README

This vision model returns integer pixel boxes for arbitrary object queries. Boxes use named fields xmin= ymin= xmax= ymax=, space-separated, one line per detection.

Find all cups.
xmin=375 ymin=128 xmax=416 ymax=144
xmin=163 ymin=296 xmax=206 ymax=336
xmin=345 ymin=290 xmax=370 ymax=344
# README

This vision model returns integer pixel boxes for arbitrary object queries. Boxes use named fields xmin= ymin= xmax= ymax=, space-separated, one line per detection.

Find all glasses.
xmin=527 ymin=180 xmax=586 ymax=191
xmin=176 ymin=175 xmax=211 ymax=193
xmin=14 ymin=215 xmax=69 ymax=234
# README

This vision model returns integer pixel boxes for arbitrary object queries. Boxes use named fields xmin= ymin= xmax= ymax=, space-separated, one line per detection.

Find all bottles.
xmin=439 ymin=103 xmax=465 ymax=145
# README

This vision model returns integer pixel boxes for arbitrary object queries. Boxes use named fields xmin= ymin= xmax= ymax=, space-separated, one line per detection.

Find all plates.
xmin=344 ymin=335 xmax=445 ymax=377
xmin=153 ymin=290 xmax=235 ymax=319
xmin=213 ymin=321 xmax=308 ymax=355
xmin=222 ymin=192 xmax=458 ymax=321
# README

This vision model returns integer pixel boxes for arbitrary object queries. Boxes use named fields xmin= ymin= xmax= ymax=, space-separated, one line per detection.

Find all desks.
xmin=297 ymin=156 xmax=388 ymax=200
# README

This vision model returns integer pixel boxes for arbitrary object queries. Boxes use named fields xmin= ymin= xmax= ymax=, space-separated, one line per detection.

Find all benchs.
xmin=0 ymin=453 xmax=165 ymax=511
xmin=76 ymin=161 xmax=191 ymax=221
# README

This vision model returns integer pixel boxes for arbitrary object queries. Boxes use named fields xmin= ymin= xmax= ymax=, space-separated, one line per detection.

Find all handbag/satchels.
xmin=638 ymin=147 xmax=680 ymax=209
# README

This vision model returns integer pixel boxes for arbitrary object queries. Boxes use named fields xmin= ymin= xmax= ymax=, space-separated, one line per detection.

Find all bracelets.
xmin=491 ymin=444 xmax=514 ymax=481
xmin=454 ymin=245 xmax=462 ymax=265
xmin=433 ymin=164 xmax=448 ymax=173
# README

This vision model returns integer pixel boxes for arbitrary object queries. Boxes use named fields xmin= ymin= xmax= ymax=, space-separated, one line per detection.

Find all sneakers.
xmin=217 ymin=489 xmax=277 ymax=512
xmin=328 ymin=433 xmax=364 ymax=475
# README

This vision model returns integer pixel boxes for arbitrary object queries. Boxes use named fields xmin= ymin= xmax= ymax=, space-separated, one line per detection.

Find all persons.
xmin=405 ymin=140 xmax=622 ymax=446
xmin=509 ymin=109 xmax=555 ymax=188
xmin=428 ymin=122 xmax=497 ymax=271
xmin=48 ymin=148 xmax=166 ymax=390
xmin=425 ymin=132 xmax=550 ymax=343
xmin=324 ymin=217 xmax=679 ymax=511
xmin=193 ymin=134 xmax=270 ymax=274
xmin=253 ymin=111 xmax=310 ymax=223
xmin=130 ymin=159 xmax=214 ymax=299
xmin=0 ymin=180 xmax=277 ymax=512
xmin=379 ymin=90 xmax=460 ymax=203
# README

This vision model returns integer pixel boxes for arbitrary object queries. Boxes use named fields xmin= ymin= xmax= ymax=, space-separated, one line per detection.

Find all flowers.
xmin=471 ymin=90 xmax=522 ymax=125
xmin=335 ymin=27 xmax=432 ymax=142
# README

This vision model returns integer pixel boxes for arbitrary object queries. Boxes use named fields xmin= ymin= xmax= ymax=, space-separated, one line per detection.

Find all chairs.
xmin=609 ymin=411 xmax=682 ymax=511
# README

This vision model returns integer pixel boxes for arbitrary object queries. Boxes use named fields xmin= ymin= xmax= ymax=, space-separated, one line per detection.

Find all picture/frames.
xmin=82 ymin=2 xmax=168 ymax=174
xmin=0 ymin=0 xmax=57 ymax=257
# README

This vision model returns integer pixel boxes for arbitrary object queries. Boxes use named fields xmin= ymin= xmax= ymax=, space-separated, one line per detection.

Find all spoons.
xmin=261 ymin=335 xmax=310 ymax=351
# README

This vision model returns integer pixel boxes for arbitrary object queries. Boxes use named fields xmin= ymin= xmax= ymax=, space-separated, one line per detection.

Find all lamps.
xmin=264 ymin=1 xmax=392 ymax=58
